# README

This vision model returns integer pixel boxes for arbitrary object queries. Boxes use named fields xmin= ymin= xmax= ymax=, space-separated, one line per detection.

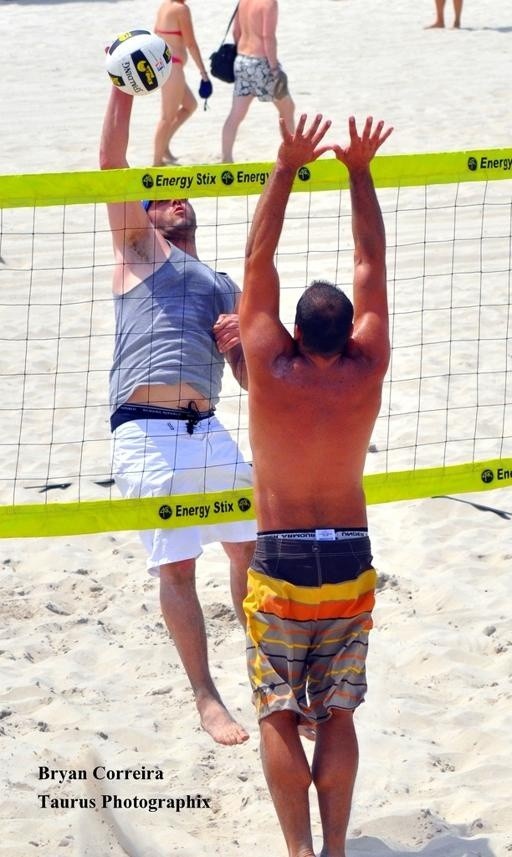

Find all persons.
xmin=263 ymin=126 xmax=265 ymax=129
xmin=423 ymin=0 xmax=466 ymax=32
xmin=149 ymin=1 xmax=213 ymax=168
xmin=232 ymin=110 xmax=399 ymax=857
xmin=100 ymin=44 xmax=261 ymax=746
xmin=220 ymin=0 xmax=297 ymax=164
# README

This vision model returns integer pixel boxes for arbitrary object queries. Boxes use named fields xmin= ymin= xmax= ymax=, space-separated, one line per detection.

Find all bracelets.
xmin=200 ymin=70 xmax=208 ymax=76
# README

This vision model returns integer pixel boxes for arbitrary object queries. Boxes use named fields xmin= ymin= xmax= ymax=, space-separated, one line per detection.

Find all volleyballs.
xmin=106 ymin=29 xmax=172 ymax=96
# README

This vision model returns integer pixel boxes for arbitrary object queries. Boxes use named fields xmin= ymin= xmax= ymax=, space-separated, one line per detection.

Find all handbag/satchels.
xmin=210 ymin=43 xmax=237 ymax=84
